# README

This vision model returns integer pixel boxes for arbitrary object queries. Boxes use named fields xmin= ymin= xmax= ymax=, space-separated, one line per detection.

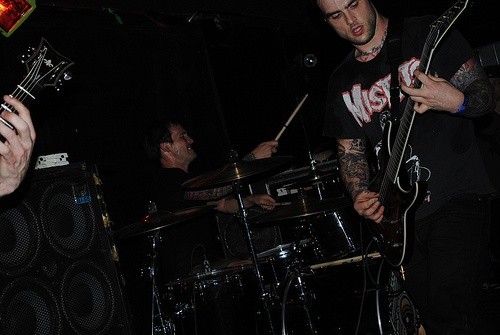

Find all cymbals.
xmin=267 ymin=159 xmax=340 ymax=186
xmin=181 ymin=156 xmax=292 ymax=190
xmin=112 ymin=204 xmax=207 ymax=238
xmin=248 ymin=198 xmax=353 ymax=225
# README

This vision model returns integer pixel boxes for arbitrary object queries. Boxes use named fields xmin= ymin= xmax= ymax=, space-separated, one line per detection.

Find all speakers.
xmin=0 ymin=161 xmax=137 ymax=335
xmin=213 ymin=204 xmax=284 ymax=261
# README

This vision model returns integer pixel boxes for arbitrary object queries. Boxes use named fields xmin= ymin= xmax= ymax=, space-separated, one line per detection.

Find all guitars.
xmin=365 ymin=0 xmax=468 ymax=266
xmin=0 ymin=36 xmax=75 ymax=139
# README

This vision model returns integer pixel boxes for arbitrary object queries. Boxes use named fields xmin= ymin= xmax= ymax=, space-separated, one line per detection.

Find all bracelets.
xmin=450 ymin=95 xmax=469 ymax=115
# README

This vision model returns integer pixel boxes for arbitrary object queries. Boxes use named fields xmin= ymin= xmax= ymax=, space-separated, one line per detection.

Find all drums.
xmin=165 ymin=264 xmax=257 ymax=335
xmin=256 ymin=237 xmax=329 ymax=285
xmin=281 ymin=258 xmax=420 ymax=335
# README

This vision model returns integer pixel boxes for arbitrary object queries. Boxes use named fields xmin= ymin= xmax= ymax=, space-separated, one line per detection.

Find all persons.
xmin=145 ymin=116 xmax=279 ymax=274
xmin=0 ymin=94 xmax=36 ymax=197
xmin=315 ymin=0 xmax=496 ymax=335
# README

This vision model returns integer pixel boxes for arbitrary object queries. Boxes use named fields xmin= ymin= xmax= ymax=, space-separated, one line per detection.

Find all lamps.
xmin=292 ymin=54 xmax=317 ymax=69
